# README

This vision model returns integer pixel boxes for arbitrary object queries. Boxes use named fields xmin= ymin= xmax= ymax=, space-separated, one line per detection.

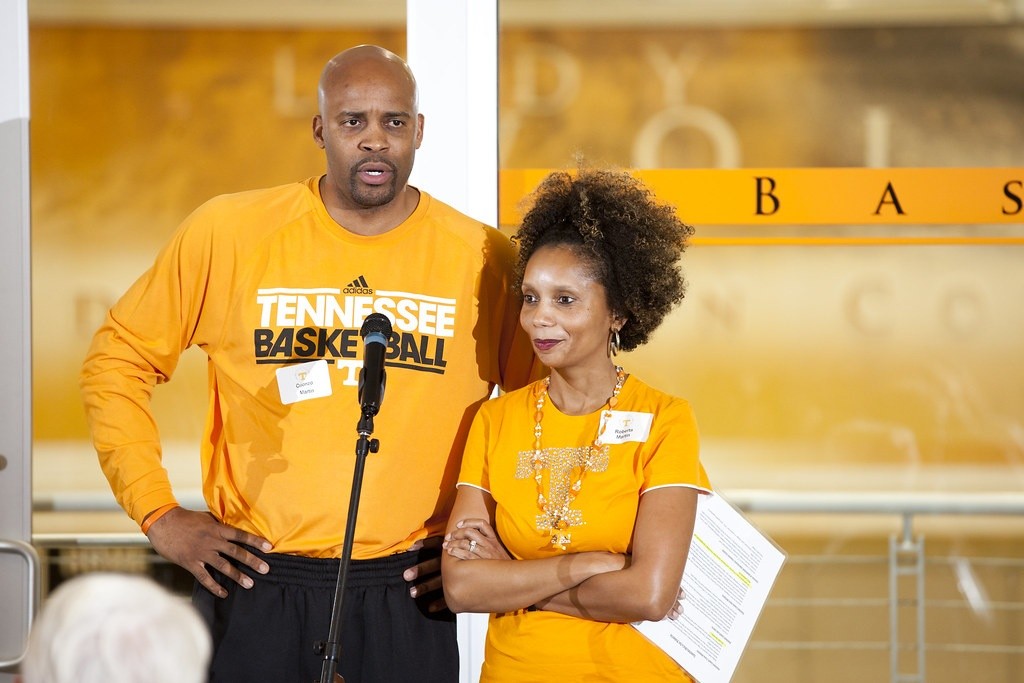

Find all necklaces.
xmin=531 ymin=363 xmax=627 ymax=550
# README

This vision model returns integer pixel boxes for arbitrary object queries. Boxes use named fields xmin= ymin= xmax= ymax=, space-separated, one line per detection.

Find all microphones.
xmin=359 ymin=313 xmax=393 ymax=419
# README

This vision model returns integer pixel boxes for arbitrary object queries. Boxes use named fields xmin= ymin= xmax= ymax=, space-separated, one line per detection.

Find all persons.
xmin=79 ymin=43 xmax=550 ymax=683
xmin=438 ymin=151 xmax=714 ymax=683
